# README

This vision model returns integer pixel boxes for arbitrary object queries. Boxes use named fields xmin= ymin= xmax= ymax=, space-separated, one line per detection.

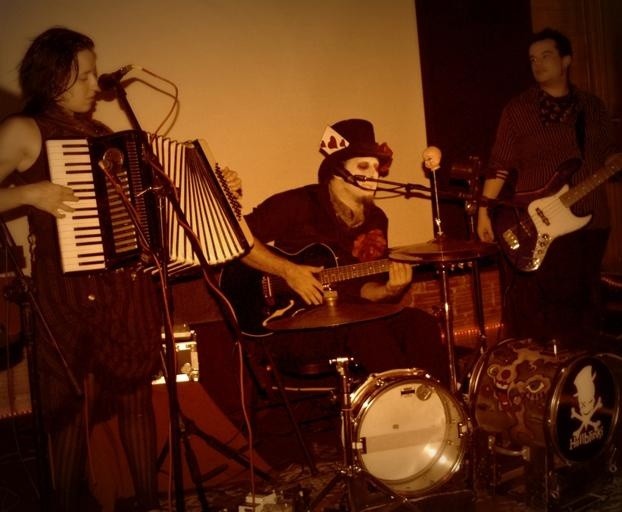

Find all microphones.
xmin=101 ymin=149 xmax=123 ymax=172
xmin=97 ymin=63 xmax=134 ymax=90
xmin=353 ymin=173 xmax=406 ymax=188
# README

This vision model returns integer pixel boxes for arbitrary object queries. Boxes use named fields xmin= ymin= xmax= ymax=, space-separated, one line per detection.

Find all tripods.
xmin=303 ymin=385 xmax=418 ymax=512
xmin=108 ymin=183 xmax=274 ymax=512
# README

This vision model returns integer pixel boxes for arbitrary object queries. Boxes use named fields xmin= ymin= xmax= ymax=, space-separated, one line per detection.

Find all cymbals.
xmin=262 ymin=300 xmax=405 ymax=334
xmin=389 ymin=239 xmax=502 ymax=264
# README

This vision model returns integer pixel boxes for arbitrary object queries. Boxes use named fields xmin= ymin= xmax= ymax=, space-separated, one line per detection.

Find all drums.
xmin=467 ymin=337 xmax=622 ymax=466
xmin=340 ymin=370 xmax=467 ymax=495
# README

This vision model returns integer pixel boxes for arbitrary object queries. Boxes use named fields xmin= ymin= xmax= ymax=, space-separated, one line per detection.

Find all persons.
xmin=0 ymin=28 xmax=242 ymax=512
xmin=236 ymin=119 xmax=440 ymax=374
xmin=476 ymin=27 xmax=622 ymax=340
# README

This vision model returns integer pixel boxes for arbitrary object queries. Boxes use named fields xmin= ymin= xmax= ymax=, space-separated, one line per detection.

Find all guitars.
xmin=218 ymin=242 xmax=473 ymax=334
xmin=488 ymin=150 xmax=621 ymax=273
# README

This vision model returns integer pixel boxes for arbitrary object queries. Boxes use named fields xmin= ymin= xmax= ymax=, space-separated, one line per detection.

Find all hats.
xmin=318 ymin=119 xmax=393 ymax=187
